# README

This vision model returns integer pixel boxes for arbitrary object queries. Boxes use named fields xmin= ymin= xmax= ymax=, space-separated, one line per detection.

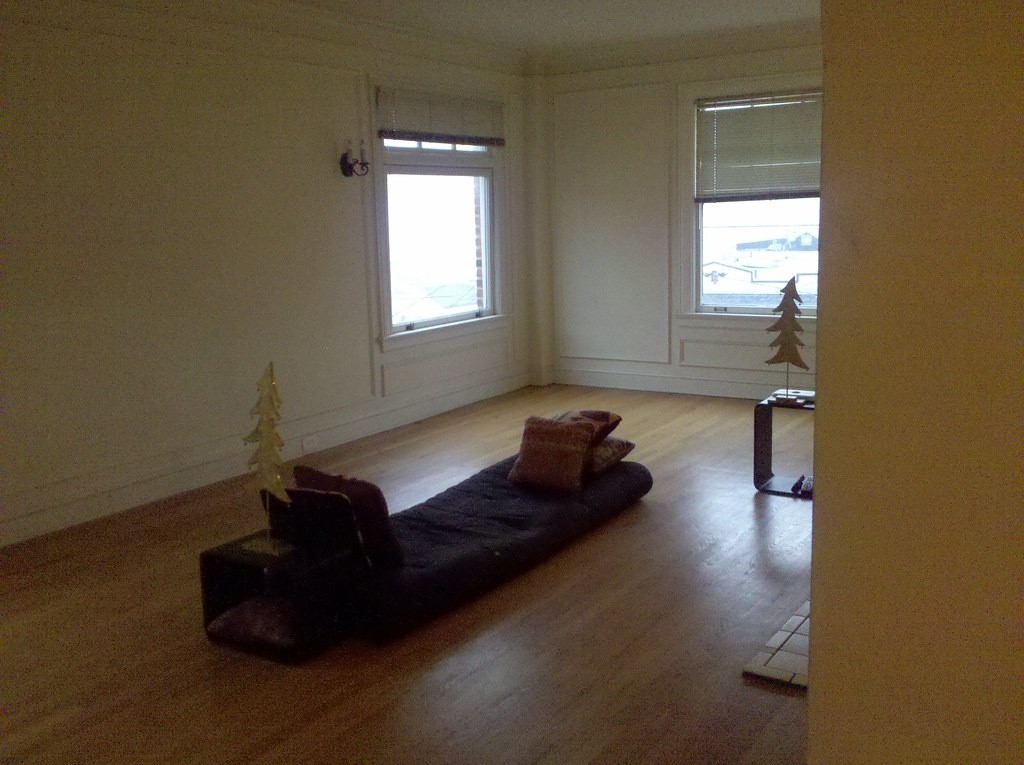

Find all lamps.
xmin=340 ymin=140 xmax=369 ymax=177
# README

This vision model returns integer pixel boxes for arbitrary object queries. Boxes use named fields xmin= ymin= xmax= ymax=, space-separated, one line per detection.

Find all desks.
xmin=753 ymin=395 xmax=814 ymax=499
xmin=199 ymin=528 xmax=354 ymax=664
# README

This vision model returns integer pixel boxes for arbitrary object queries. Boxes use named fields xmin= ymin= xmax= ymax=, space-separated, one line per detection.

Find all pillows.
xmin=553 ymin=410 xmax=623 ymax=446
xmin=585 ymin=436 xmax=636 ymax=476
xmin=507 ymin=414 xmax=594 ymax=495
xmin=257 ymin=464 xmax=405 ymax=585
